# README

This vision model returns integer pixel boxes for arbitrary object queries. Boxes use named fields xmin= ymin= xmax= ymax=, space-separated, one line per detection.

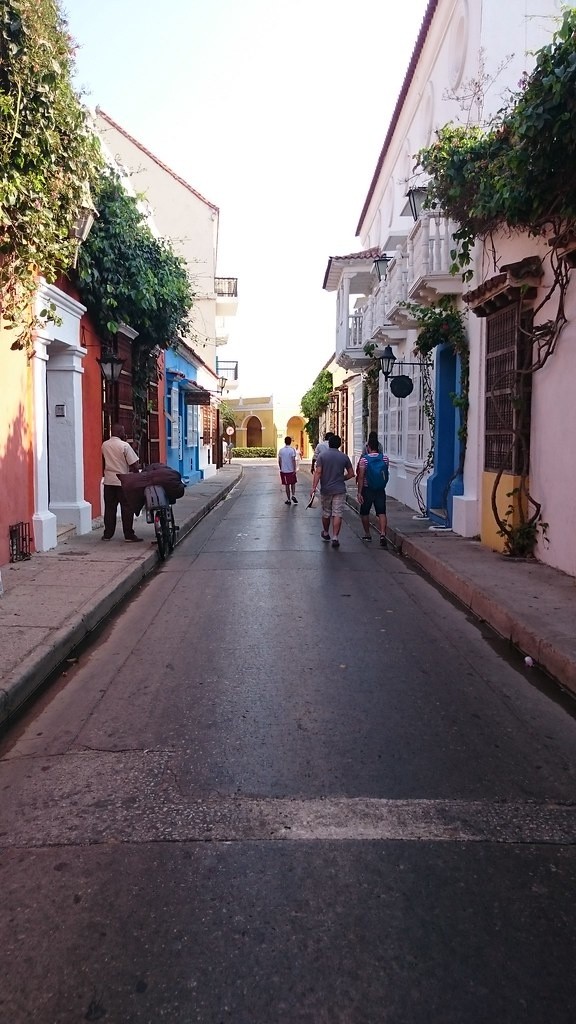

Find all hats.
xmin=305 ymin=492 xmax=320 ymax=510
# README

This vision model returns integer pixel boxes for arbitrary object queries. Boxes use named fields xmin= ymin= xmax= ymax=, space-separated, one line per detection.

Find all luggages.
xmin=144 ymin=485 xmax=169 ymax=510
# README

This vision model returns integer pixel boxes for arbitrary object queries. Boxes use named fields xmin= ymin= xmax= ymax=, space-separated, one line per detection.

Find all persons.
xmin=357 ymin=438 xmax=389 ymax=541
xmin=356 ymin=431 xmax=383 ymax=473
xmin=294 ymin=445 xmax=302 ymax=470
xmin=223 ymin=438 xmax=228 ymax=464
xmin=311 ymin=432 xmax=334 ymax=474
xmin=311 ymin=435 xmax=354 ymax=548
xmin=278 ymin=436 xmax=298 ymax=505
xmin=102 ymin=424 xmax=143 ymax=541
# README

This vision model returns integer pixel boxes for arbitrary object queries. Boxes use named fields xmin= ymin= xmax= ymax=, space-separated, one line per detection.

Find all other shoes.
xmin=101 ymin=535 xmax=111 ymax=541
xmin=285 ymin=500 xmax=291 ymax=505
xmin=380 ymin=535 xmax=387 ymax=546
xmin=292 ymin=496 xmax=298 ymax=503
xmin=124 ymin=535 xmax=143 ymax=542
xmin=361 ymin=536 xmax=372 ymax=542
xmin=332 ymin=538 xmax=340 ymax=547
xmin=321 ymin=531 xmax=331 ymax=541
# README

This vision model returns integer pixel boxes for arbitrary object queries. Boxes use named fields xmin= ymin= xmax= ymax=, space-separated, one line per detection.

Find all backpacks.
xmin=363 ymin=453 xmax=389 ymax=491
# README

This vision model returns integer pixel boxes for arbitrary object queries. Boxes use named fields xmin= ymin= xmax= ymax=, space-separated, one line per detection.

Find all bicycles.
xmin=144 ymin=484 xmax=188 ymax=560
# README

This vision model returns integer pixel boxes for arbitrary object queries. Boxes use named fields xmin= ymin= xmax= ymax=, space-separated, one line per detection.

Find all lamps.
xmin=405 ymin=185 xmax=434 ymax=220
xmin=374 ymin=253 xmax=393 ymax=281
xmin=203 ymin=376 xmax=228 ymax=396
xmin=380 ymin=345 xmax=435 ymax=382
xmin=82 ymin=339 xmax=127 ymax=384
xmin=70 ymin=180 xmax=100 ymax=268
